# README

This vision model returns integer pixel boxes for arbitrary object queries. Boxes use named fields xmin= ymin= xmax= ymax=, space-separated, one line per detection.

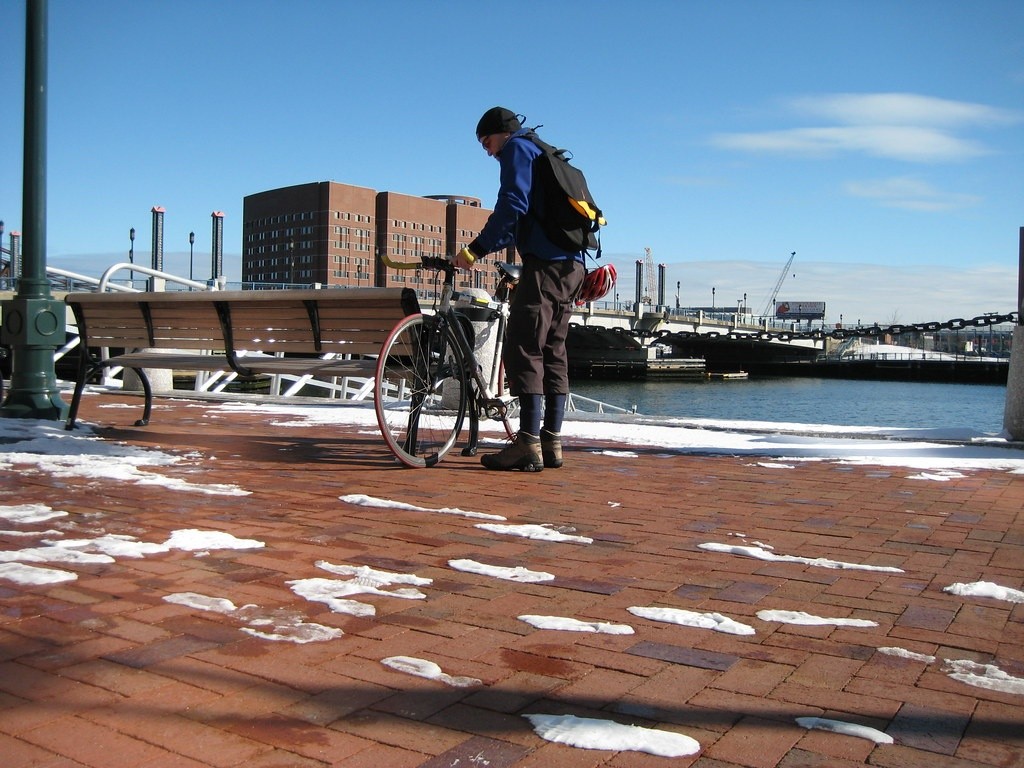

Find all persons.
xmin=450 ymin=106 xmax=587 ymax=473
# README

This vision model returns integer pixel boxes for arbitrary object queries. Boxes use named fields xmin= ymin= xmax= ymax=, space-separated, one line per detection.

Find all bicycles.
xmin=373 ymin=251 xmax=521 ymax=468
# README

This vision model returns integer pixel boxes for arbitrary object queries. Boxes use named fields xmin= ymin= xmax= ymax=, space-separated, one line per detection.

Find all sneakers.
xmin=540 ymin=426 xmax=563 ymax=468
xmin=481 ymin=430 xmax=544 ymax=472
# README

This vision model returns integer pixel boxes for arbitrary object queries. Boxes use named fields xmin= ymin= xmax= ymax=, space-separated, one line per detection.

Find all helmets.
xmin=575 ymin=264 xmax=617 ymax=306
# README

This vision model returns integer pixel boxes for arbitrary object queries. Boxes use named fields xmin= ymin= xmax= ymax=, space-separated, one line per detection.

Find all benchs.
xmin=65 ymin=287 xmax=479 ymax=464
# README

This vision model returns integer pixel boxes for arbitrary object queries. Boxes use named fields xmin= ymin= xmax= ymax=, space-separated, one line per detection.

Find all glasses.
xmin=482 ymin=135 xmax=491 ymax=150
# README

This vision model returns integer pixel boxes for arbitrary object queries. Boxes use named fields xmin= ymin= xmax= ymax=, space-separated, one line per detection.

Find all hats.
xmin=476 ymin=106 xmax=522 ymax=143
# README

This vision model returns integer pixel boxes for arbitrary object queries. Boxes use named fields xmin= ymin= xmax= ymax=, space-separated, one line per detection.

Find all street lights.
xmin=129 ymin=228 xmax=136 ymax=279
xmin=676 ymin=280 xmax=680 ymax=297
xmin=840 ymin=315 xmax=842 ymax=328
xmin=773 ymin=299 xmax=776 ymax=317
xmin=799 ymin=305 xmax=802 ymax=324
xmin=983 ymin=312 xmax=997 ymax=352
xmin=712 ymin=287 xmax=715 ymax=319
xmin=0 ymin=220 xmax=4 ymax=289
xmin=190 ymin=230 xmax=196 ymax=292
xmin=744 ymin=293 xmax=747 ymax=314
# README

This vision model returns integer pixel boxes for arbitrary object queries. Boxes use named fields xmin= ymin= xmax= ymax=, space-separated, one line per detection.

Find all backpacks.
xmin=501 ymin=134 xmax=607 ymax=251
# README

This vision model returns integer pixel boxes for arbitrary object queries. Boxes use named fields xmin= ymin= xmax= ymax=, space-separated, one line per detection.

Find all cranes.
xmin=758 ymin=251 xmax=797 ymax=326
xmin=644 ymin=247 xmax=658 ymax=306
